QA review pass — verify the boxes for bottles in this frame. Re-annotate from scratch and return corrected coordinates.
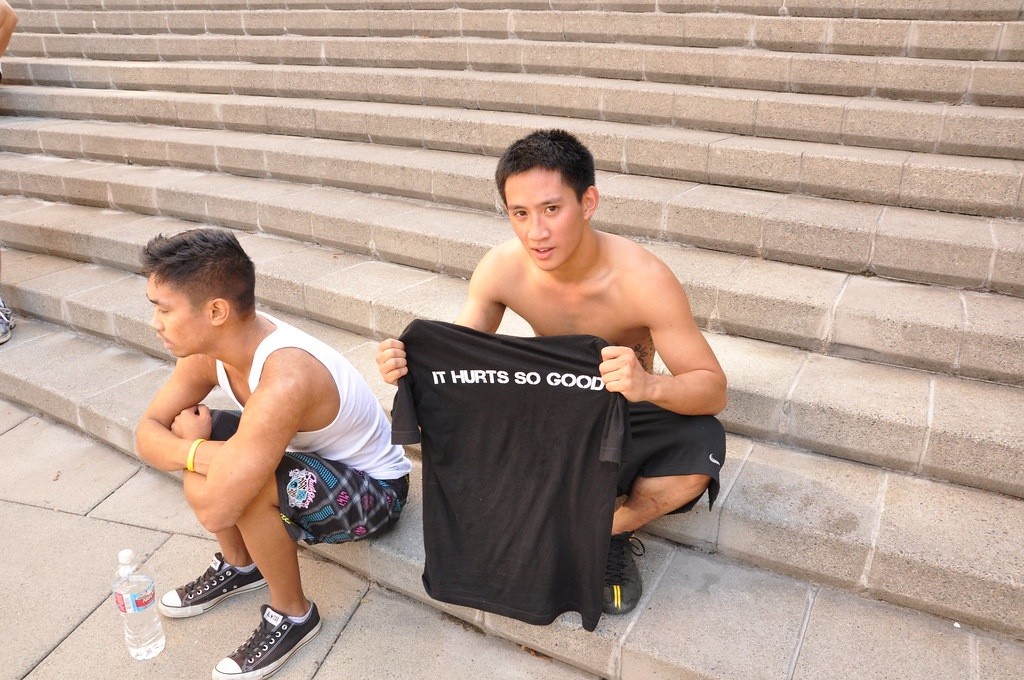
[115,548,164,660]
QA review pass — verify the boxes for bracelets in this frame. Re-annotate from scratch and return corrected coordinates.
[188,438,206,471]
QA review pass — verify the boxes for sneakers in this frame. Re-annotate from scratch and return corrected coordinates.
[212,601,321,680]
[159,552,268,618]
[601,530,645,615]
[0,297,15,344]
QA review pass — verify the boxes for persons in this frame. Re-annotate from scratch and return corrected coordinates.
[135,227,409,680]
[377,128,728,615]
[0,1,20,346]
